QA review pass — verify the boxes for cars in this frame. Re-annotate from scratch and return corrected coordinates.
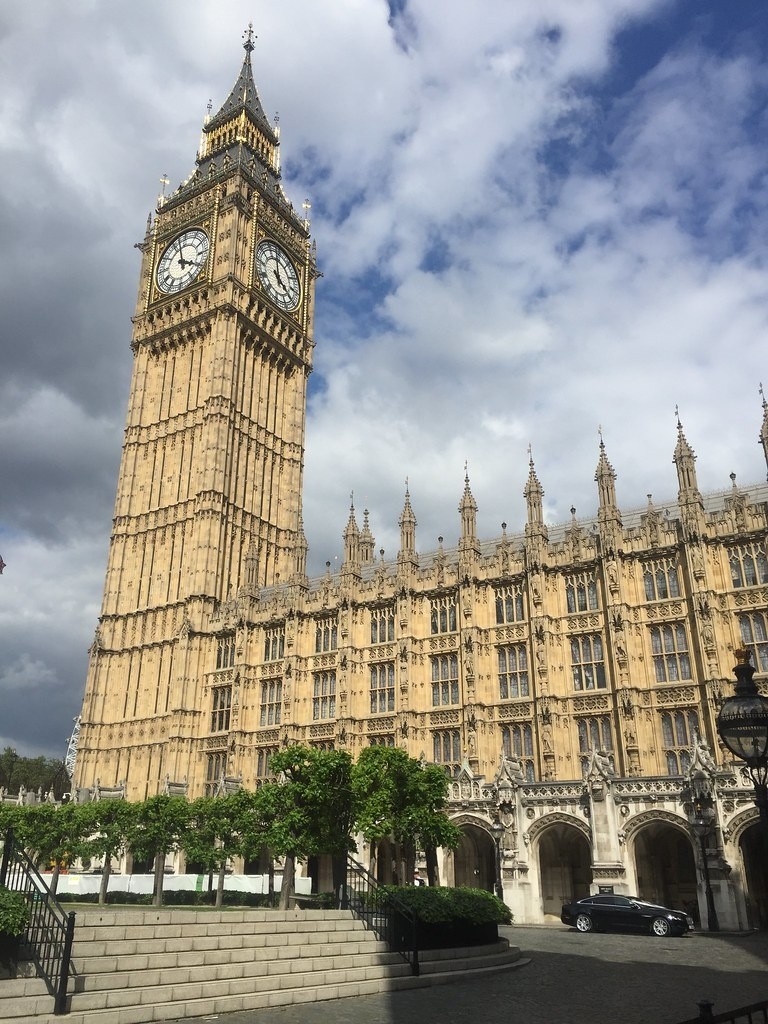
[559,893,695,939]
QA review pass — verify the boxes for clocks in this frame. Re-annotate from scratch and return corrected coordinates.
[153,226,210,294]
[254,236,302,313]
